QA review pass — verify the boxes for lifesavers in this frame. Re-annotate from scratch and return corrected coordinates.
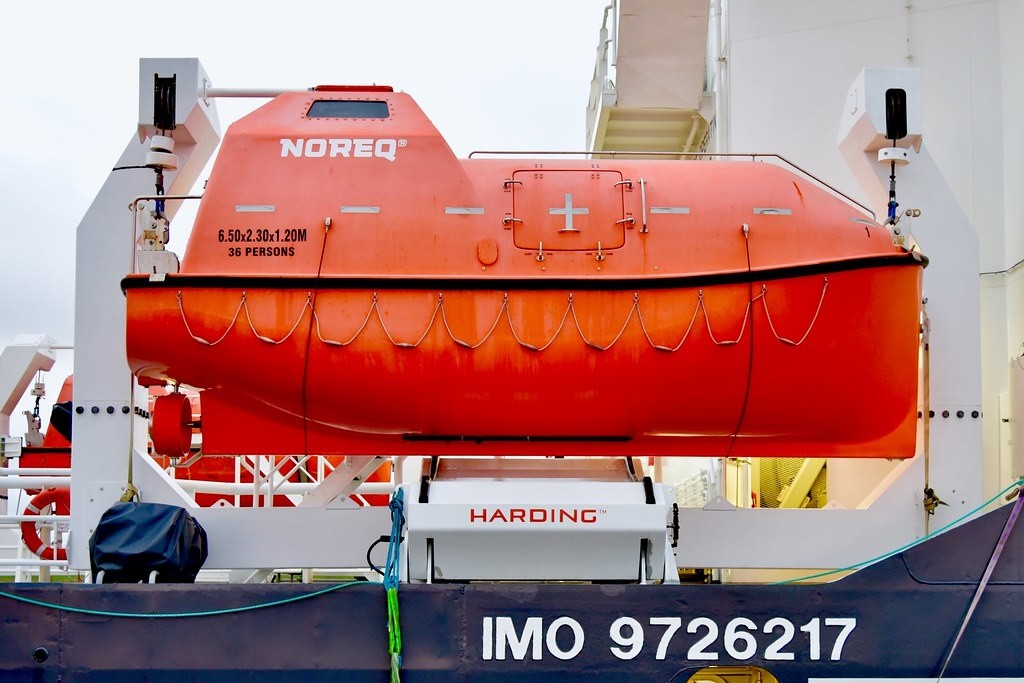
[21,489,70,560]
